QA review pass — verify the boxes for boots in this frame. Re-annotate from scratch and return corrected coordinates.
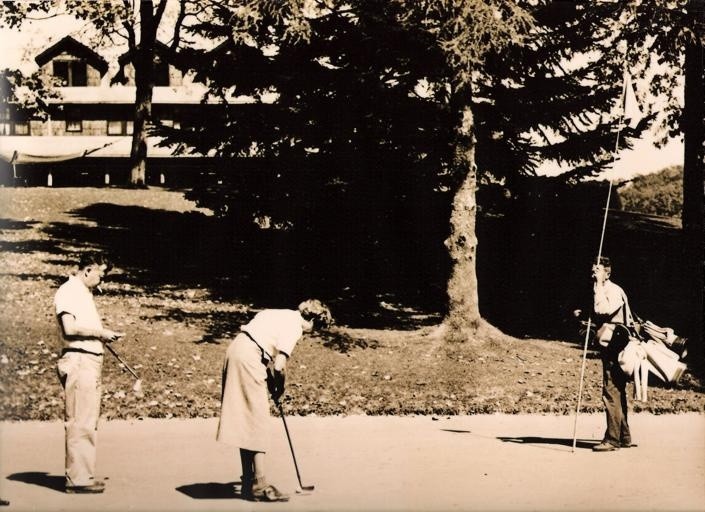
[240,475,291,502]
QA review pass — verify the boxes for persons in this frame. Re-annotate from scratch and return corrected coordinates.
[574,256,637,452]
[216,299,332,501]
[53,250,128,494]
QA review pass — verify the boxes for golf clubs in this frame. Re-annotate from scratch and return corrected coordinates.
[266,367,313,490]
[103,341,142,392]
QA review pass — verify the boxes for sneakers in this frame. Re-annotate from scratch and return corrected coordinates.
[66,480,105,493]
[592,442,631,451]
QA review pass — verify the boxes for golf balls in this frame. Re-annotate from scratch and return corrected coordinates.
[296,489,301,493]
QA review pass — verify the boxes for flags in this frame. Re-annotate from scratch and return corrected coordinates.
[622,76,643,121]
